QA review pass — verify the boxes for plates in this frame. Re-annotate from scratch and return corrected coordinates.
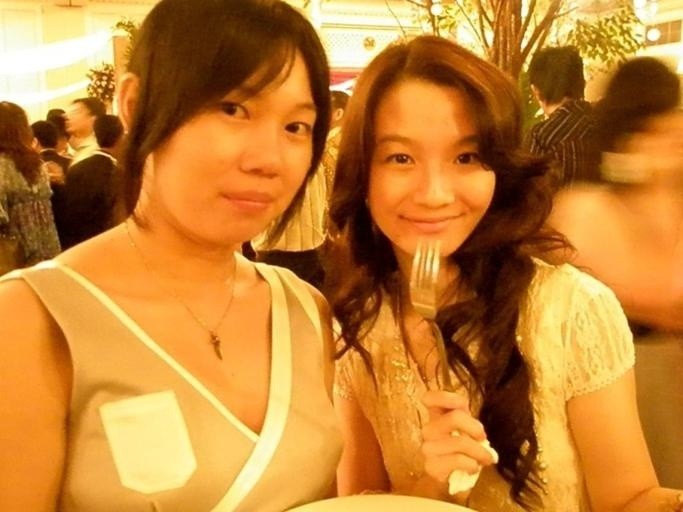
[288,495,476,512]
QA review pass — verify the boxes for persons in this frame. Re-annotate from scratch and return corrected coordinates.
[0,0,347,512]
[0,46,682,511]
[316,34,681,511]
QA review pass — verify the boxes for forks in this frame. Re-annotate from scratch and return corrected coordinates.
[407,237,455,396]
